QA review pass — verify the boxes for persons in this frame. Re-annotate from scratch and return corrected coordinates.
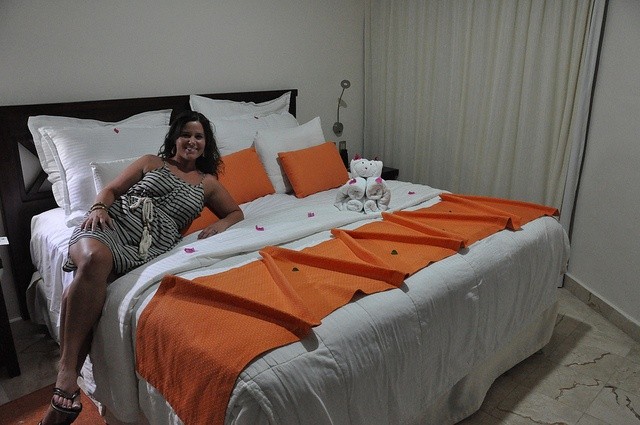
[38,111,244,425]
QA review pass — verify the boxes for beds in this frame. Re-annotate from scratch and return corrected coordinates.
[0,88,571,425]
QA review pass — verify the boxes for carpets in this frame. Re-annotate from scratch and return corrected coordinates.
[0,381,109,425]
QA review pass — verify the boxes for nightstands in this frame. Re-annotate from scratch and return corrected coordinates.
[1,251,25,383]
[343,160,402,182]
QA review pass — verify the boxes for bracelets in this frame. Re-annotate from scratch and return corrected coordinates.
[90,202,110,211]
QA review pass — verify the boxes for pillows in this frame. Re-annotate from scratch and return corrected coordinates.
[212,147,276,205]
[255,116,326,194]
[37,125,171,229]
[90,157,142,194]
[181,206,220,238]
[278,142,349,198]
[27,109,172,209]
[189,92,300,154]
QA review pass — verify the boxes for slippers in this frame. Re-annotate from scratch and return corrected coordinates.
[51,387,83,413]
